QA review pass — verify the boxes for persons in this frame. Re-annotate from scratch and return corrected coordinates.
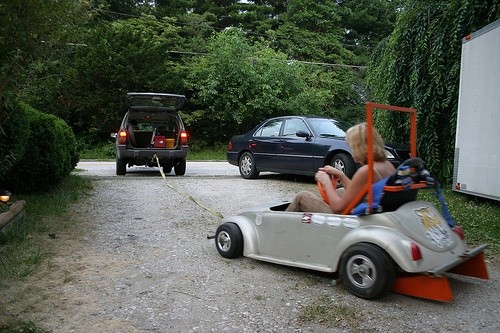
[285,121,399,217]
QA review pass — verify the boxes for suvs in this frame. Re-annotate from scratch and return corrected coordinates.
[111,92,188,176]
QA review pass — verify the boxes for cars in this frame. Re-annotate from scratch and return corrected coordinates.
[206,189,488,302]
[226,116,402,189]
